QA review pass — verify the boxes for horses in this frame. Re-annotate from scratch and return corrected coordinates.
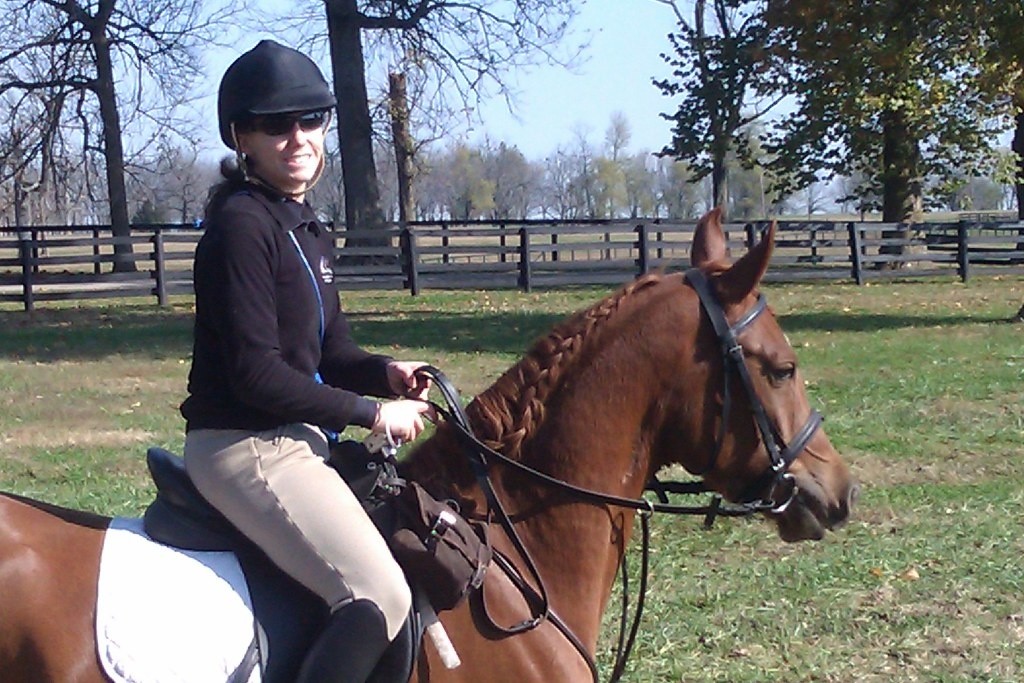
[0,207,857,683]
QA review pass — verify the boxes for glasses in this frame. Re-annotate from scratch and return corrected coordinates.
[234,106,331,137]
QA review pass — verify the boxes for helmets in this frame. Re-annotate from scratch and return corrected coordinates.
[216,39,338,152]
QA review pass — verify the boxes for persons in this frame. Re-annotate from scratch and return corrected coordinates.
[182,40,433,683]
